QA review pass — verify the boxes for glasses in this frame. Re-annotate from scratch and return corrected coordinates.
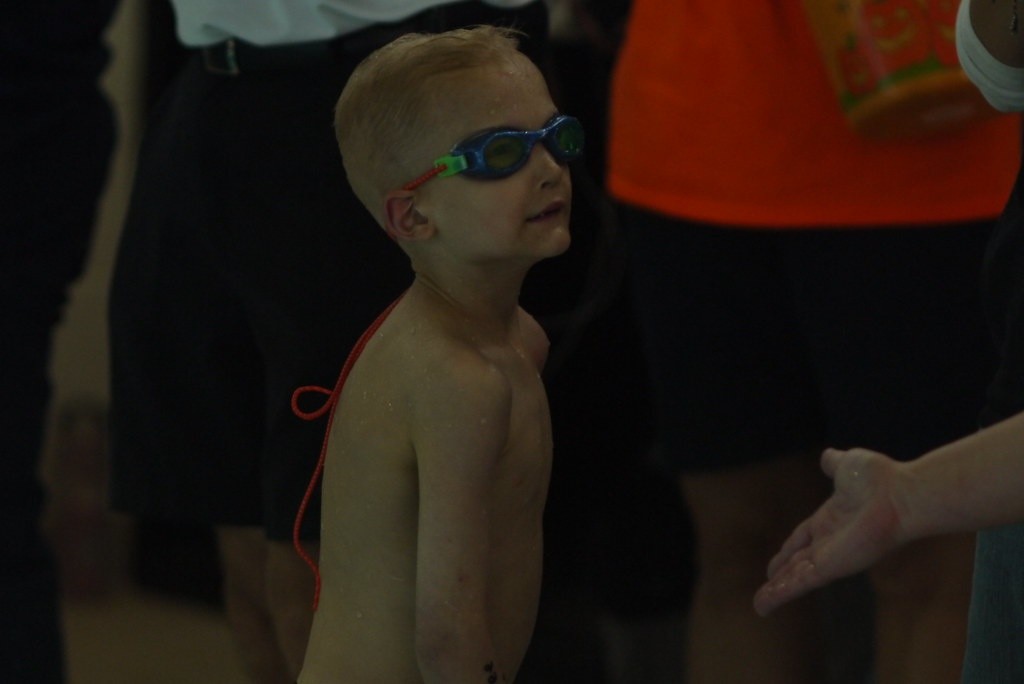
[400,112,588,192]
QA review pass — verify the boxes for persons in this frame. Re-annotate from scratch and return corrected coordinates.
[285,23,583,684]
[598,3,1023,684]
[1,1,551,684]
[751,0,1023,684]
